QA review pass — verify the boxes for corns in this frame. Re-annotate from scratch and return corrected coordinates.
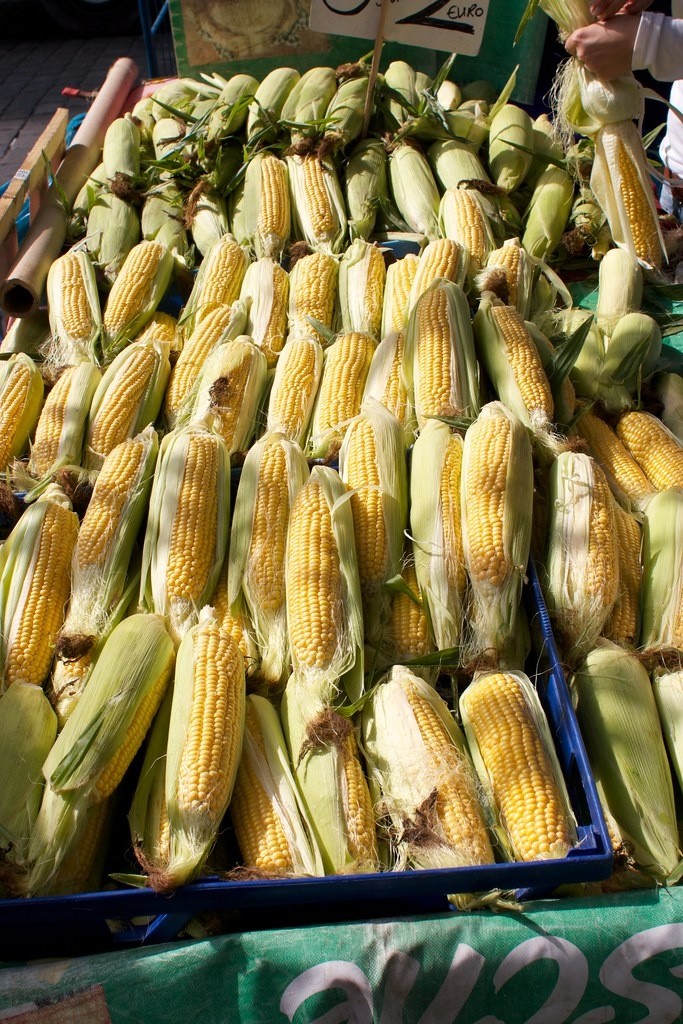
[0,64,683,894]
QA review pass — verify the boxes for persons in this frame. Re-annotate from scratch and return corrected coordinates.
[564,1,682,224]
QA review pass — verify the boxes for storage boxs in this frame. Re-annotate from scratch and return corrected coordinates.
[0,107,421,509]
[0,554,614,966]
